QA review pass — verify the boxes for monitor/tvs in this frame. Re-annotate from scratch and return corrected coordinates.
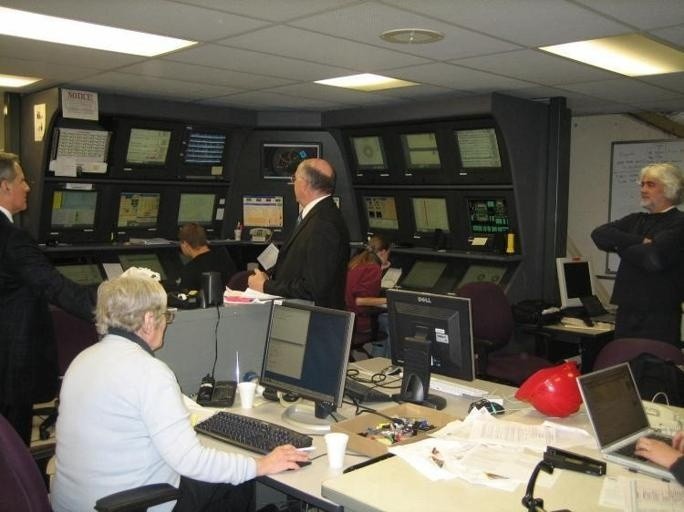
[241,193,285,229]
[556,255,597,309]
[258,298,356,431]
[387,289,475,410]
[50,124,228,288]
[349,128,519,294]
[260,142,323,182]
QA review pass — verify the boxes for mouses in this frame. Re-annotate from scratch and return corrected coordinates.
[382,365,402,375]
[287,461,312,471]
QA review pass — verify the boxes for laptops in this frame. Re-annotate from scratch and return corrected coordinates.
[575,361,676,479]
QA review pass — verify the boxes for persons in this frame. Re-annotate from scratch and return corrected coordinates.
[248,158,350,314]
[592,163,684,350]
[179,221,237,291]
[48,266,311,512]
[635,428,683,486]
[345,235,393,357]
[0,151,96,450]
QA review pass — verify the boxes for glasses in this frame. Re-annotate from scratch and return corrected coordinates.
[156,310,176,324]
[290,175,314,184]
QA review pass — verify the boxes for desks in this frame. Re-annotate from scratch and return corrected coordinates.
[179,354,539,512]
[321,393,684,512]
[516,316,615,374]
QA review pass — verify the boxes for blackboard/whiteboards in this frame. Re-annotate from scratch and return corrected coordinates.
[605,139,684,274]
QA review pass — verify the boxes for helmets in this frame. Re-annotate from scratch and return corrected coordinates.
[513,360,583,417]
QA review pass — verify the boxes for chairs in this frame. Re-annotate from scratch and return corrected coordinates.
[590,337,684,374]
[0,412,183,512]
[227,270,257,292]
[351,308,387,363]
[24,307,99,439]
[453,281,557,386]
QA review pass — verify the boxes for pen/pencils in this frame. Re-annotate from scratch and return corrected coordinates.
[627,468,670,482]
[236,221,243,229]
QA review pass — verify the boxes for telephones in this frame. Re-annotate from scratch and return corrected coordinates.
[250,227,272,243]
[197,373,238,407]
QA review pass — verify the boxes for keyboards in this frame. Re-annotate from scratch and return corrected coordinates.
[428,374,488,400]
[194,411,313,455]
[344,378,391,402]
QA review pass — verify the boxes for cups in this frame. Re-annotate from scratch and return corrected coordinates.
[237,382,258,413]
[234,230,243,240]
[325,432,350,472]
